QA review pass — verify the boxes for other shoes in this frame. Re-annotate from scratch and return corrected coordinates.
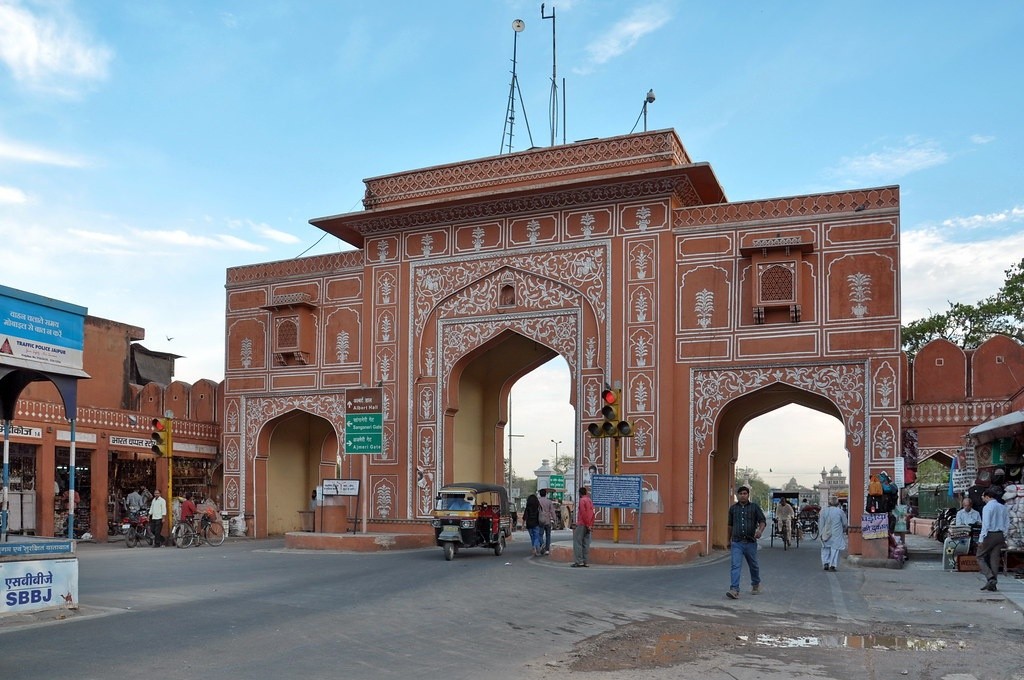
[536,553,541,556]
[545,551,549,555]
[751,585,758,595]
[829,566,836,571]
[532,547,536,553]
[570,563,589,567]
[980,580,997,592]
[726,591,739,599]
[824,564,829,570]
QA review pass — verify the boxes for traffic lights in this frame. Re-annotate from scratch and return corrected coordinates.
[587,388,635,439]
[151,418,168,458]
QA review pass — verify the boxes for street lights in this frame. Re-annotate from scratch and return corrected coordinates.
[551,439,562,466]
[508,18,526,153]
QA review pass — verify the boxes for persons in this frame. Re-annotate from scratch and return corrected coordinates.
[140,484,153,507]
[956,498,982,542]
[147,489,167,548]
[776,497,794,546]
[819,497,847,571]
[126,486,144,515]
[62,490,80,510]
[799,499,810,512]
[841,505,848,519]
[311,490,317,508]
[570,487,595,567]
[976,490,1010,591]
[176,492,206,548]
[449,494,472,510]
[538,490,557,554]
[726,487,766,599]
[521,494,542,556]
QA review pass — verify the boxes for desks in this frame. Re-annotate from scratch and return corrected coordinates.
[1000,550,1024,576]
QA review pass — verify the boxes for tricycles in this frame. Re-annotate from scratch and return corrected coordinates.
[770,491,800,550]
[799,504,821,540]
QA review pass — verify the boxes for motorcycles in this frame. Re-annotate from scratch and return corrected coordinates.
[123,508,154,549]
[431,482,511,561]
[936,507,956,543]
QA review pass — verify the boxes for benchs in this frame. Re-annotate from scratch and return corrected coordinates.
[472,505,501,534]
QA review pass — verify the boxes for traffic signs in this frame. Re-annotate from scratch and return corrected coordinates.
[345,414,383,433]
[346,389,383,414]
[345,434,383,455]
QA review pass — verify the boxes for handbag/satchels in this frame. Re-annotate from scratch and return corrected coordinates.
[538,505,546,526]
[821,518,832,542]
[866,473,898,513]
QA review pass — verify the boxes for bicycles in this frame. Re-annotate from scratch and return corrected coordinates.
[172,509,225,549]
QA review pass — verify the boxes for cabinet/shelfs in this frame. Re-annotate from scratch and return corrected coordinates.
[77,475,206,492]
